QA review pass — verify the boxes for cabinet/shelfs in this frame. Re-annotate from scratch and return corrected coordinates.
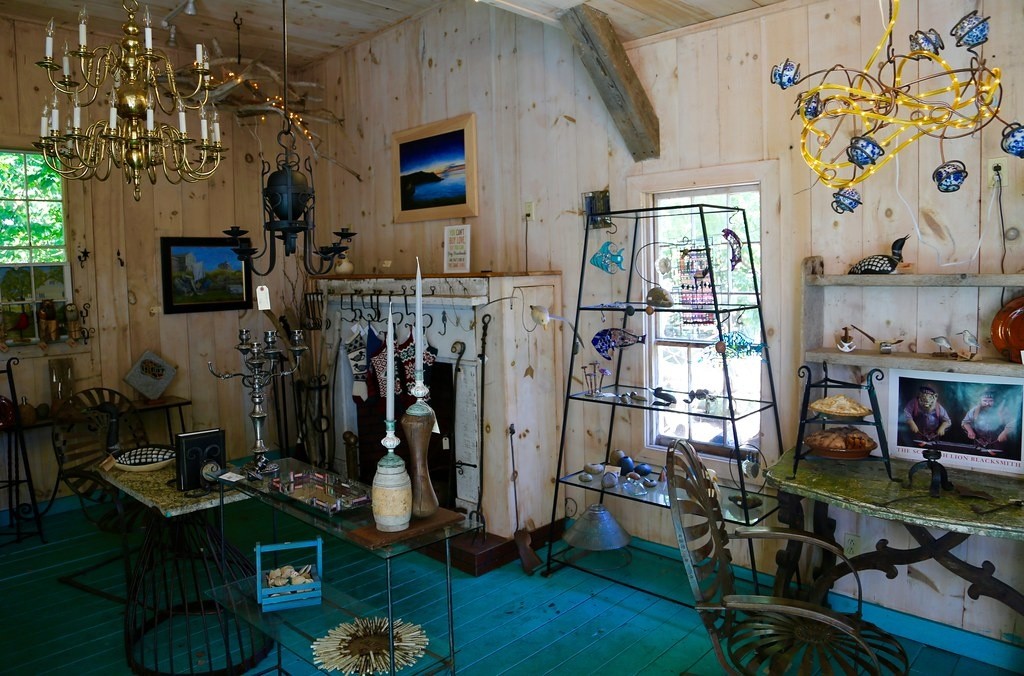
[204,457,484,676]
[798,252,1024,455]
[540,203,804,625]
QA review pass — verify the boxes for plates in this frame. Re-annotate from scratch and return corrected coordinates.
[110,444,176,471]
[5,338,41,346]
[802,440,878,460]
[808,405,874,421]
[990,296,1024,364]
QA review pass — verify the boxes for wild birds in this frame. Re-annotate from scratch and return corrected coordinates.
[849,233,911,275]
[955,329,982,353]
[930,335,954,353]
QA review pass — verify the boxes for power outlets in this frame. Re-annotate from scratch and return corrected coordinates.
[844,532,862,559]
[986,156,1009,188]
[525,202,535,222]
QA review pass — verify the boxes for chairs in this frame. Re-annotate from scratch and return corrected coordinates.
[49,387,261,615]
[666,438,910,676]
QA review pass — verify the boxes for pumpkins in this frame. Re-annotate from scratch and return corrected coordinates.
[19,396,36,426]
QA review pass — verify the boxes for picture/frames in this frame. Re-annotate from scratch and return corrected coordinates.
[159,235,253,315]
[390,112,479,225]
[890,367,1024,474]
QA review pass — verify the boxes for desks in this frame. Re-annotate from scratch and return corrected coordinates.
[762,443,1024,655]
[85,445,280,676]
[0,392,194,545]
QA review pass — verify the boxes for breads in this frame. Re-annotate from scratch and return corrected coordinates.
[805,425,876,451]
[809,395,873,416]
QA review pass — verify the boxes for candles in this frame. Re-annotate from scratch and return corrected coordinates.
[386,303,395,422]
[415,256,423,371]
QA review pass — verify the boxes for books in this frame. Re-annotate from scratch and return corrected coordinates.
[176,428,226,489]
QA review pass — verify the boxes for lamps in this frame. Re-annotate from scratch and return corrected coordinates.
[27,0,230,202]
[221,0,357,276]
